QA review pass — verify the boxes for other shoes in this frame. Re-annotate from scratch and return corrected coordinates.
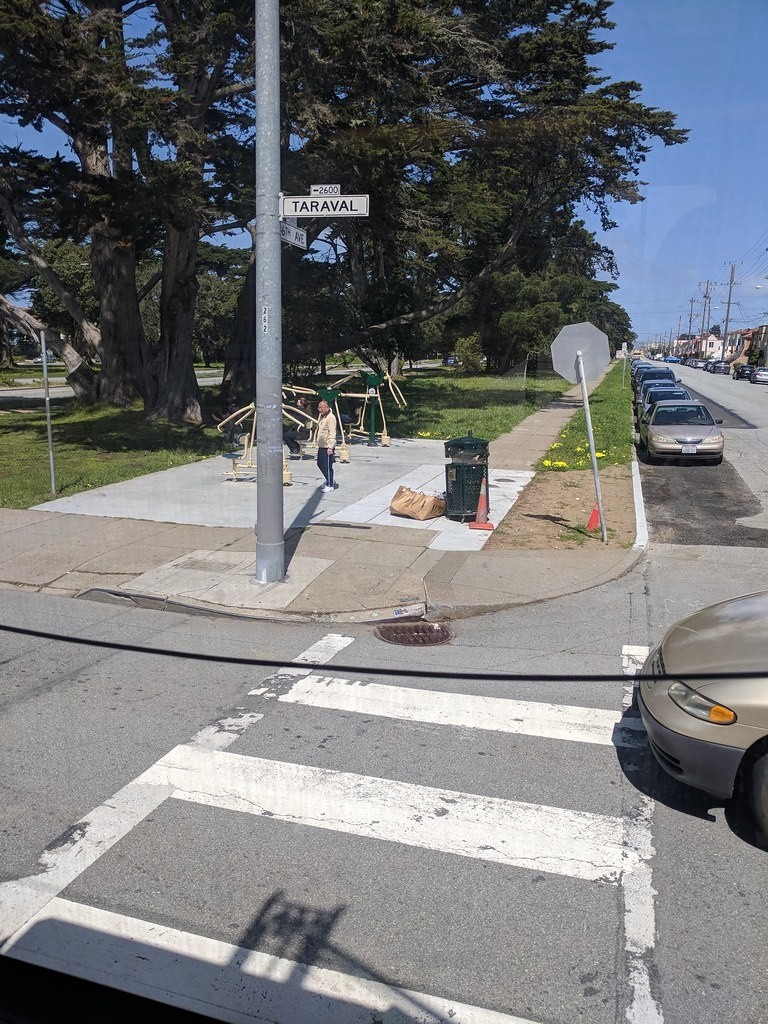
[288,447,301,454]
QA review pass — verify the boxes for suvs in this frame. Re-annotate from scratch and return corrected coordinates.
[665,357,681,364]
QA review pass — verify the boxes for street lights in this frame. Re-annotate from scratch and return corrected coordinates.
[696,321,709,359]
[715,307,730,360]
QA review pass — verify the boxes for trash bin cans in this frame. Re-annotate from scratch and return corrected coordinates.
[443,437,490,524]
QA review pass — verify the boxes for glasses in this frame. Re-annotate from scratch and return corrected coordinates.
[317,406,328,411]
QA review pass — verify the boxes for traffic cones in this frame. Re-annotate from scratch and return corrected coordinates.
[469,478,494,530]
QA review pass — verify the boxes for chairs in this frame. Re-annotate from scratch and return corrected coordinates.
[342,406,362,439]
[294,420,314,460]
[223,433,250,482]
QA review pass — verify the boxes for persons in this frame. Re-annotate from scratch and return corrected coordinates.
[284,398,311,454]
[224,401,246,446]
[317,402,337,492]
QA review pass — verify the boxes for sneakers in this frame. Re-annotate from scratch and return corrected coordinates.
[320,486,335,493]
[319,481,327,489]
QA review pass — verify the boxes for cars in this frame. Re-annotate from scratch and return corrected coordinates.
[751,367,768,384]
[630,353,700,433]
[710,360,731,375]
[733,365,756,380]
[442,357,458,367]
[640,400,724,464]
[638,590,768,852]
[685,359,715,372]
[33,354,56,364]
